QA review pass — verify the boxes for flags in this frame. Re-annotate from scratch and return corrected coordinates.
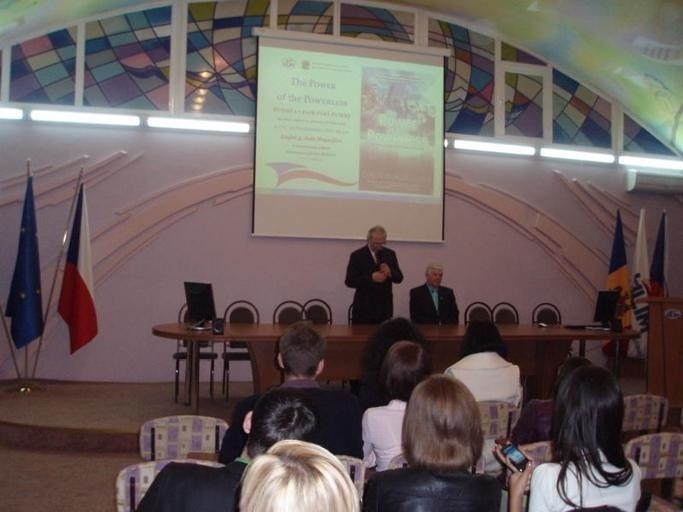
[649,211,665,295]
[627,209,648,358]
[607,209,632,332]
[56,182,98,356]
[3,175,42,350]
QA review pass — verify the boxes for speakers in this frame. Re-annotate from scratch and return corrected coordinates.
[212,317,225,336]
[611,319,623,333]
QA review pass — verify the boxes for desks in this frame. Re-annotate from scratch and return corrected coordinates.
[152,324,641,400]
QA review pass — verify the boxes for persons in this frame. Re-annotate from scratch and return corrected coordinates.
[133,386,320,512]
[495,364,642,512]
[443,315,523,411]
[219,320,363,465]
[345,224,403,323]
[236,440,361,512]
[363,374,502,512]
[360,340,434,471]
[510,357,592,443]
[408,262,460,325]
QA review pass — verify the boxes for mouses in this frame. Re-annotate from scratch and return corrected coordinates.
[539,322,548,328]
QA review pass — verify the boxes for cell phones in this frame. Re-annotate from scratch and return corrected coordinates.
[500,444,529,471]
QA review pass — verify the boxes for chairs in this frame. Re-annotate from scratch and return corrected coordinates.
[464,302,493,324]
[342,303,353,388]
[173,302,217,405]
[300,298,332,384]
[532,302,561,324]
[222,300,259,401]
[491,302,520,324]
[273,300,308,324]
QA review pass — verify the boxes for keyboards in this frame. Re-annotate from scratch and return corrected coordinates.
[564,324,602,329]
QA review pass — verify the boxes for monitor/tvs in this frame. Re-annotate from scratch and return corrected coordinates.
[592,290,620,328]
[183,281,216,330]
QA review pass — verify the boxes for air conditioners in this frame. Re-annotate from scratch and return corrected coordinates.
[625,169,683,194]
